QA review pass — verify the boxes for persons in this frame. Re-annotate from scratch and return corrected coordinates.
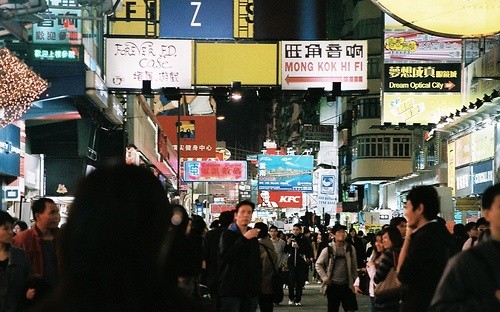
[21,164,210,312]
[314,224,363,312]
[301,226,313,285]
[267,225,286,307]
[315,233,330,284]
[372,225,407,312]
[460,216,490,252]
[254,221,279,312]
[165,203,190,292]
[185,91,218,116]
[396,183,458,311]
[283,232,290,290]
[389,216,408,241]
[13,220,29,236]
[278,229,285,239]
[283,223,314,306]
[200,210,234,311]
[182,213,206,301]
[430,183,500,311]
[208,219,220,231]
[348,227,362,291]
[215,199,263,311]
[352,231,386,311]
[466,220,477,236]
[258,191,278,208]
[381,222,390,228]
[310,231,323,284]
[356,229,368,254]
[1,210,34,312]
[12,196,65,281]
[451,223,468,243]
[446,219,456,235]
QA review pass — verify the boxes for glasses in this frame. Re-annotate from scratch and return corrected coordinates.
[478,228,487,232]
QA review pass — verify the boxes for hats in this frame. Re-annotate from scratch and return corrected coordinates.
[254,222,268,240]
[302,227,311,234]
[332,223,347,234]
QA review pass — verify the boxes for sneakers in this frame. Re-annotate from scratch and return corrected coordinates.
[296,302,301,306]
[288,299,294,306]
[305,281,309,285]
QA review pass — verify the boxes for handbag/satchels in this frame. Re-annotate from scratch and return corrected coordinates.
[373,250,402,295]
[357,268,370,296]
[272,272,284,304]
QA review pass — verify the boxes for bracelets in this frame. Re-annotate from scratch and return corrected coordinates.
[403,236,410,244]
